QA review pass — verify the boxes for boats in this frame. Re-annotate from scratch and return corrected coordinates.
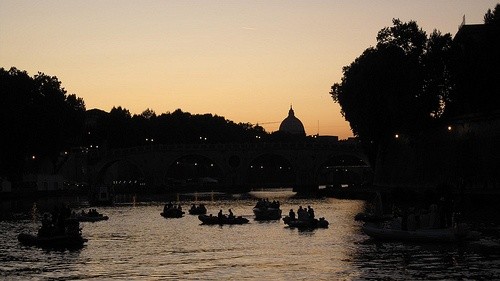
[363,222,462,241]
[188,208,207,215]
[198,214,249,224]
[78,213,103,221]
[160,210,183,218]
[252,207,281,219]
[283,217,329,229]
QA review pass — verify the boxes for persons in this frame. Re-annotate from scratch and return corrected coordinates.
[227,208,233,219]
[191,204,205,211]
[297,205,315,221]
[254,198,280,209]
[81,208,99,217]
[164,200,182,212]
[37,215,80,238]
[218,209,222,217]
[451,212,470,232]
[391,208,420,232]
[289,209,295,219]
